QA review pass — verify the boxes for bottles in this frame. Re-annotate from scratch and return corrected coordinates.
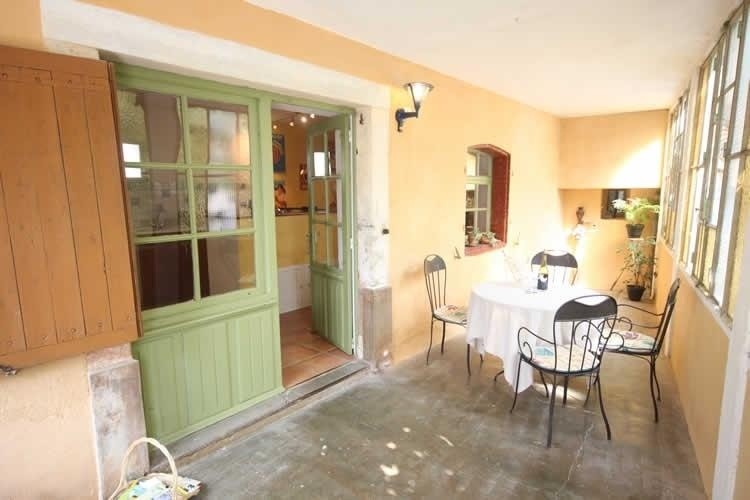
[537,253,548,290]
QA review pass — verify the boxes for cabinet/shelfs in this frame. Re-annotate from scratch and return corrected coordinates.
[277,264,312,314]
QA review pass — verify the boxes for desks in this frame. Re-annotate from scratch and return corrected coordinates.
[467,278,605,393]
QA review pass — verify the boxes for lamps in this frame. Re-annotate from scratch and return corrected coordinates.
[396,81,435,131]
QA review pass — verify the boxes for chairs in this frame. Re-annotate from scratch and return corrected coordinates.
[594,278,681,424]
[510,295,617,447]
[423,253,483,376]
[530,248,578,286]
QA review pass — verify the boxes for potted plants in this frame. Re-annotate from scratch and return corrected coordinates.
[480,232,496,243]
[616,238,658,301]
[612,198,661,238]
